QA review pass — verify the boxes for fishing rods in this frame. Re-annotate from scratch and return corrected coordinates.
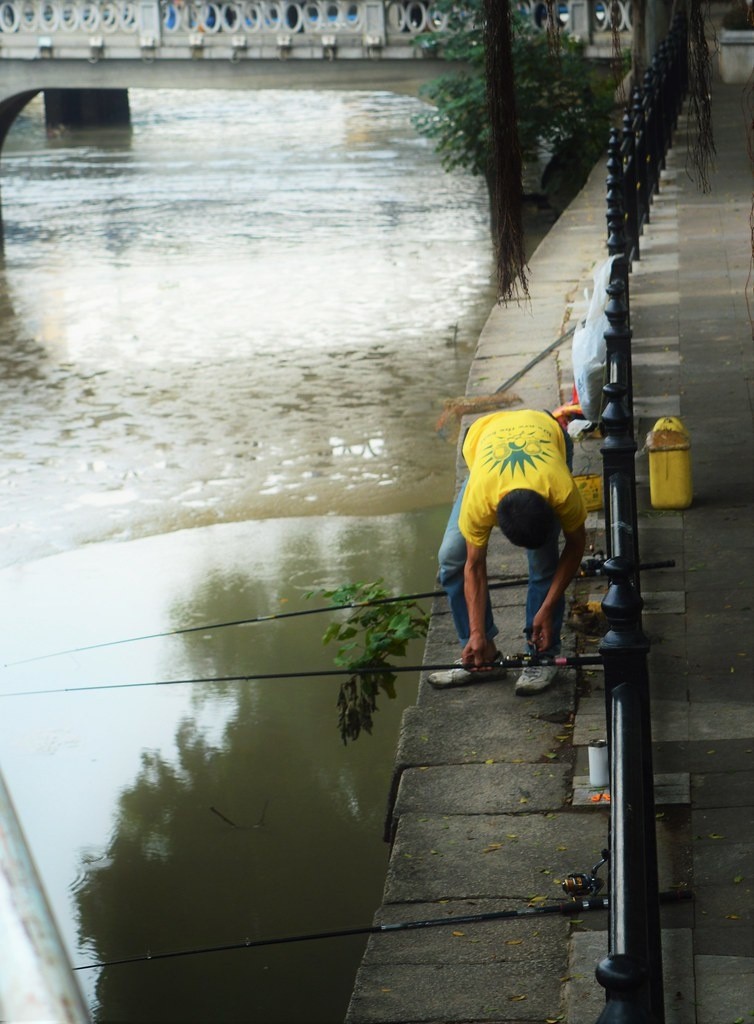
[70,850,696,973]
[0,560,678,669]
[0,651,603,698]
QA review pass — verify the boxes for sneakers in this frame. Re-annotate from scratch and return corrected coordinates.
[514,665,559,693]
[427,650,506,688]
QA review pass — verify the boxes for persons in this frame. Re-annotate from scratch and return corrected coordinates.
[421,409,589,697]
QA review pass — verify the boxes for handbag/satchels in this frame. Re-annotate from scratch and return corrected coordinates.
[571,253,625,423]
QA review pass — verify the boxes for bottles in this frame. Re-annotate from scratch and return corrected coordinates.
[649,416,693,510]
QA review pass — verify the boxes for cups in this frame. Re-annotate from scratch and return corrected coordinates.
[588,739,609,787]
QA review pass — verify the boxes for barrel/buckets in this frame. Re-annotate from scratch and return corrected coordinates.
[573,466,602,511]
